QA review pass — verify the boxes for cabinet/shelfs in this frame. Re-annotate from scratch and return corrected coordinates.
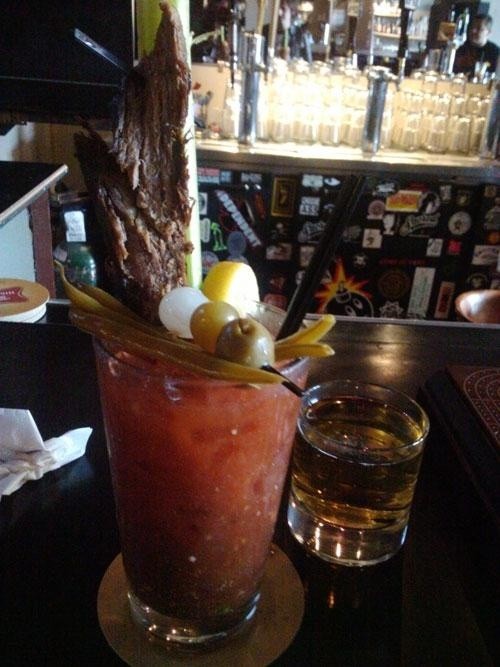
[356,0,429,53]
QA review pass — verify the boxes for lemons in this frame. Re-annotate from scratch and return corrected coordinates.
[203,260,260,302]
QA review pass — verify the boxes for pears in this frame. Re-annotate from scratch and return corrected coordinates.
[215,318,274,368]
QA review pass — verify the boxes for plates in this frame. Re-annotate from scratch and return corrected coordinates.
[97,544,309,667]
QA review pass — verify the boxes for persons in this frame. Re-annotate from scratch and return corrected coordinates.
[258,0,313,68]
[449,288,500,325]
[450,14,499,82]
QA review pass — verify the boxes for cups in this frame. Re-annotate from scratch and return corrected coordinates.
[288,380,430,571]
[88,332,313,652]
[221,54,493,155]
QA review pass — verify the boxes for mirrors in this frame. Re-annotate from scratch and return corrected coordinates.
[189,0,500,84]
